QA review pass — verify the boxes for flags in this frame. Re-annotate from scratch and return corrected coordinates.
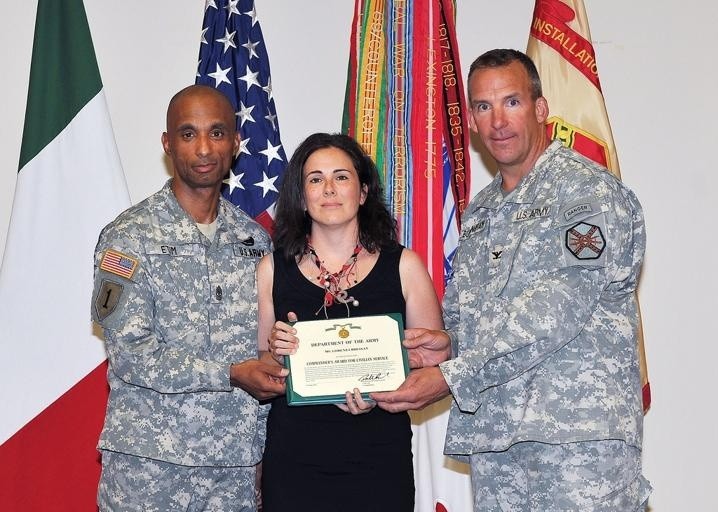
[442,0,650,475]
[340,0,472,427]
[195,1,289,237]
[1,1,135,511]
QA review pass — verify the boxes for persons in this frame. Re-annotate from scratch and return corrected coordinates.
[366,50,653,511]
[90,86,293,512]
[257,134,446,511]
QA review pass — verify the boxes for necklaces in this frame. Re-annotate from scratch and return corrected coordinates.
[305,238,365,319]
